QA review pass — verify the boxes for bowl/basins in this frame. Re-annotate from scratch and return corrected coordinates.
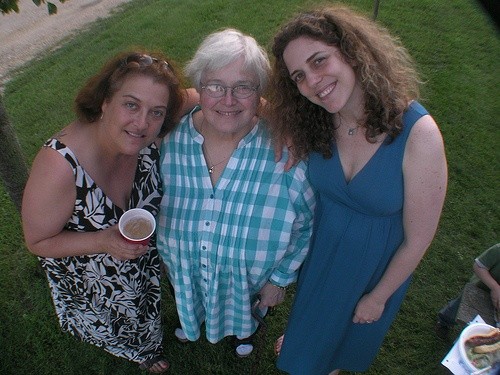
[459,323,500,375]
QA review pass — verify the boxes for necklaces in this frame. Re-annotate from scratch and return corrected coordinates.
[199,118,232,174]
[339,116,367,135]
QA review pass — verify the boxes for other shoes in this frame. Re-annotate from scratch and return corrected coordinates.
[436,318,451,340]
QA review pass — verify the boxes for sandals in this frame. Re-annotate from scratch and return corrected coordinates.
[175,324,187,339]
[234,337,254,359]
[143,357,170,374]
[274,334,285,357]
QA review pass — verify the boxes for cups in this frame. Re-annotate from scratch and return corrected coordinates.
[118,208,156,246]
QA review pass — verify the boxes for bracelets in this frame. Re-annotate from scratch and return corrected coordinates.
[272,284,290,291]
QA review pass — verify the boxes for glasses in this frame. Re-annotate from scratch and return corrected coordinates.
[199,79,261,100]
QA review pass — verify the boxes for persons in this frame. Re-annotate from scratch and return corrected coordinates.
[19,49,311,375]
[155,27,318,360]
[434,243,500,342]
[262,5,449,375]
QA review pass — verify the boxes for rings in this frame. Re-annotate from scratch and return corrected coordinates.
[366,321,370,324]
